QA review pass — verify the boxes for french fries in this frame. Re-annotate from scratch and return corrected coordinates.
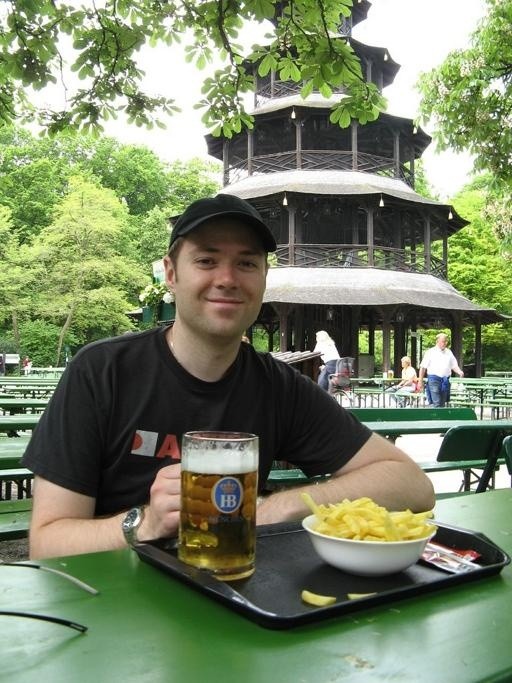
[347,592,377,600]
[302,493,438,543]
[301,590,336,607]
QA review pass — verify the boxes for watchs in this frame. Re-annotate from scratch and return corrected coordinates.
[121,503,151,546]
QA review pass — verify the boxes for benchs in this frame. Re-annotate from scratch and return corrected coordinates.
[3,497,32,544]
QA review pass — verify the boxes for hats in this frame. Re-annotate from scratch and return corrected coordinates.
[169,195,277,251]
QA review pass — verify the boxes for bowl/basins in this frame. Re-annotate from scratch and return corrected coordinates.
[302,514,438,578]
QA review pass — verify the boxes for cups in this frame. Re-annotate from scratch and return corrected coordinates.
[177,431,260,583]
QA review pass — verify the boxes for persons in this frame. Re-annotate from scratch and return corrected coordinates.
[415,331,465,438]
[385,356,418,407]
[18,193,438,561]
[24,355,30,376]
[310,329,341,392]
